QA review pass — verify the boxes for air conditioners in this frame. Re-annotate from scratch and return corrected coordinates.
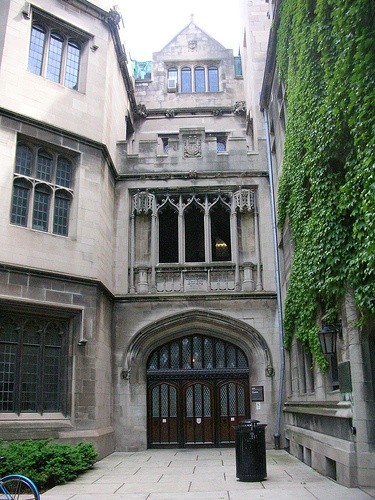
[168,77,177,93]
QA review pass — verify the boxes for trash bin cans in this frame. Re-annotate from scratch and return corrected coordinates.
[233,420,267,482]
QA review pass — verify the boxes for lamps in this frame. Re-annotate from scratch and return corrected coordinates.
[318,319,343,355]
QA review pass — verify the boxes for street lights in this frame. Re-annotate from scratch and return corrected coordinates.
[318,327,340,391]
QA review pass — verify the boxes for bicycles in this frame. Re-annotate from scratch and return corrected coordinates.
[0,456,40,500]
